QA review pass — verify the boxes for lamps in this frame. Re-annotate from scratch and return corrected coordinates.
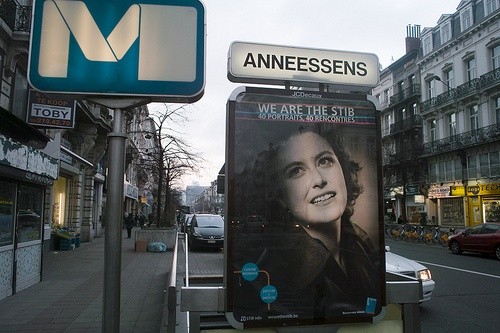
[138,151,152,156]
[130,130,152,139]
[132,147,148,152]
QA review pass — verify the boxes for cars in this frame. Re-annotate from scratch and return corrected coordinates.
[446,222,500,259]
[189,214,225,251]
[385,245,436,304]
[181,214,195,234]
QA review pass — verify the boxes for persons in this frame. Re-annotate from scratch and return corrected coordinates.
[124,209,154,238]
[177,212,180,226]
[226,122,378,323]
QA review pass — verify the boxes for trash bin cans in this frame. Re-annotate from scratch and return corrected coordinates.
[57,229,79,251]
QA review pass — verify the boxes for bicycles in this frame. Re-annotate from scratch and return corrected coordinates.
[386,222,461,247]
